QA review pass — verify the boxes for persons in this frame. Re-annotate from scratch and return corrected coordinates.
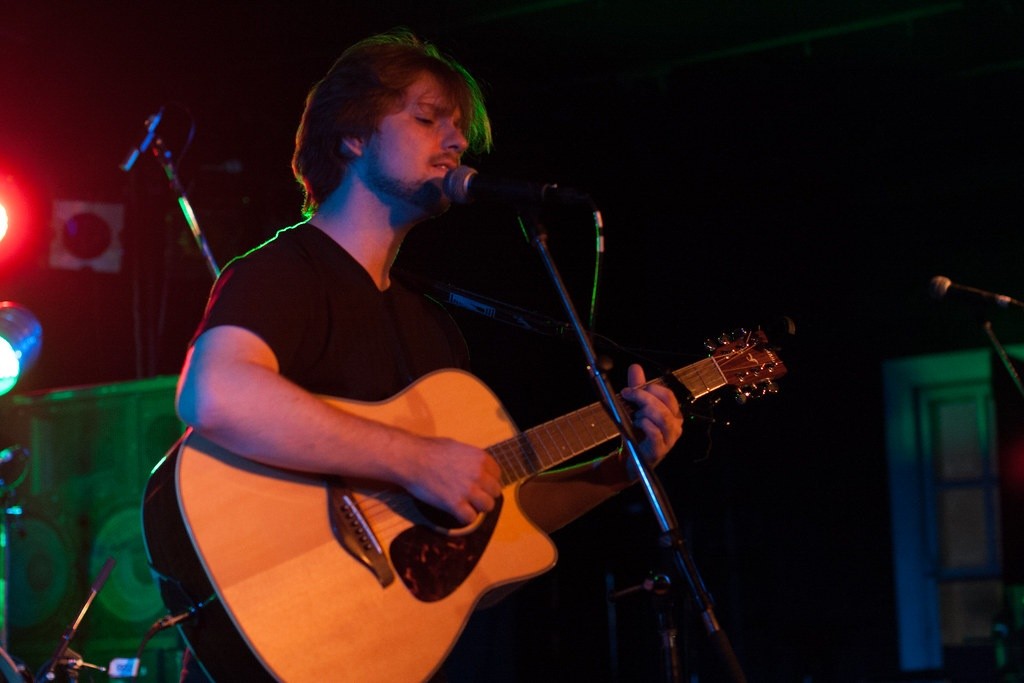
[174,32,688,683]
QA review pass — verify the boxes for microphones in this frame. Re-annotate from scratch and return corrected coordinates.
[444,168,592,207]
[119,106,166,171]
[929,275,1024,309]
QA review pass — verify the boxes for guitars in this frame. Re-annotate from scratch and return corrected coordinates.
[139,323,791,683]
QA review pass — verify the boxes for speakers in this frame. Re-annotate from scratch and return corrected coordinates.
[4,376,189,683]
